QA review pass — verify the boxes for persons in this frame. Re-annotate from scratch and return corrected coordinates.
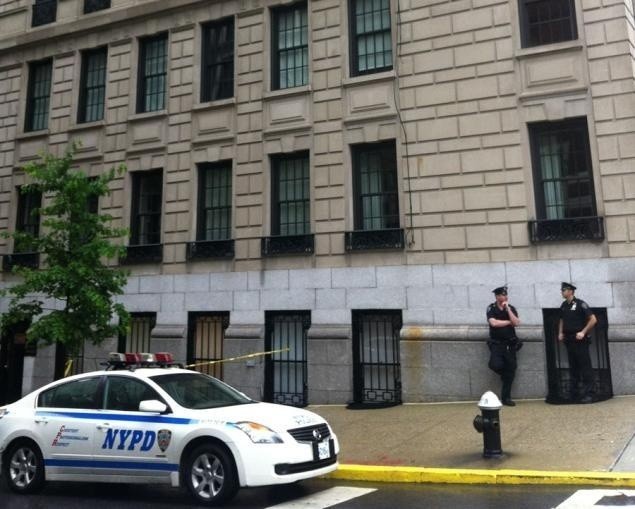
[485,286,521,406]
[558,281,597,403]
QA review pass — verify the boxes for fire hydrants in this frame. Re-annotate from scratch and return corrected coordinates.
[473,390,502,458]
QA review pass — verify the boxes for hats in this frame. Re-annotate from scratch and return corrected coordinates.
[493,287,508,295]
[561,282,576,291]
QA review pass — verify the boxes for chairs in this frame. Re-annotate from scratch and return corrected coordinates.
[55,393,99,408]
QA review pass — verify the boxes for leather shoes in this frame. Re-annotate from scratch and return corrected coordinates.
[581,395,592,402]
[502,400,515,406]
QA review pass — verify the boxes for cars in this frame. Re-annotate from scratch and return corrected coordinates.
[1,351,340,505]
